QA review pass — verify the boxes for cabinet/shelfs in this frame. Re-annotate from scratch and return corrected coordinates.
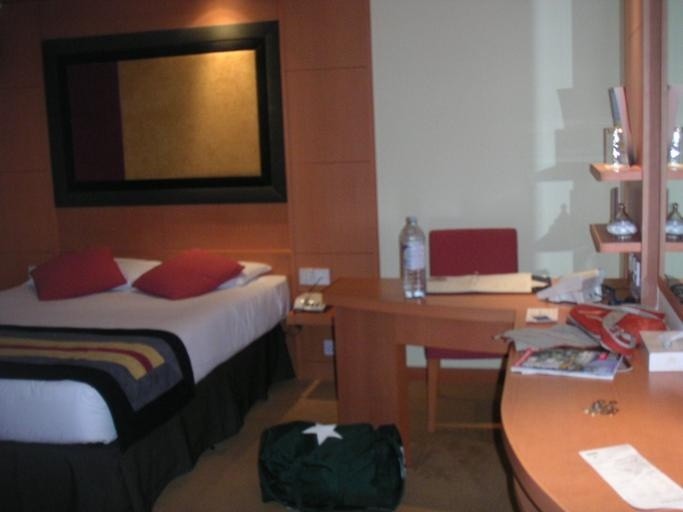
[665,165,682,251]
[589,163,642,255]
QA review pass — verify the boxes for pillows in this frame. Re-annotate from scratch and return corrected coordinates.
[127,258,272,290]
[130,250,244,300]
[25,240,126,300]
[28,257,148,292]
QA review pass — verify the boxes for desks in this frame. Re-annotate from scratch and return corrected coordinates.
[325,272,683,511]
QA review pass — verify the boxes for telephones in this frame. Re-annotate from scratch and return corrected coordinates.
[293,292,327,312]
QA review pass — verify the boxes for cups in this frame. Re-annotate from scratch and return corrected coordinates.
[603,129,623,170]
[667,128,682,166]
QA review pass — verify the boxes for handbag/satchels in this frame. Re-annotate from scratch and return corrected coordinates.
[492,301,668,353]
[259,421,405,512]
[427,273,551,294]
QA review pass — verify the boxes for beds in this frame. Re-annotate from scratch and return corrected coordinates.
[0,241,293,511]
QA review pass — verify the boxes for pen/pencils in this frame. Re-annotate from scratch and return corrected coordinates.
[514,347,534,367]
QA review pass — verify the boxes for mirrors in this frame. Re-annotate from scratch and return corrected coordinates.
[640,1,683,330]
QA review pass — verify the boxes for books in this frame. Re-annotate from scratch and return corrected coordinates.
[510,347,633,381]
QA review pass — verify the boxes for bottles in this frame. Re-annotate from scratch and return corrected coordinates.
[606,202,637,236]
[664,203,683,239]
[400,215,427,299]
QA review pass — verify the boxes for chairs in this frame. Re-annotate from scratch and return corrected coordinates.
[426,226,522,437]
